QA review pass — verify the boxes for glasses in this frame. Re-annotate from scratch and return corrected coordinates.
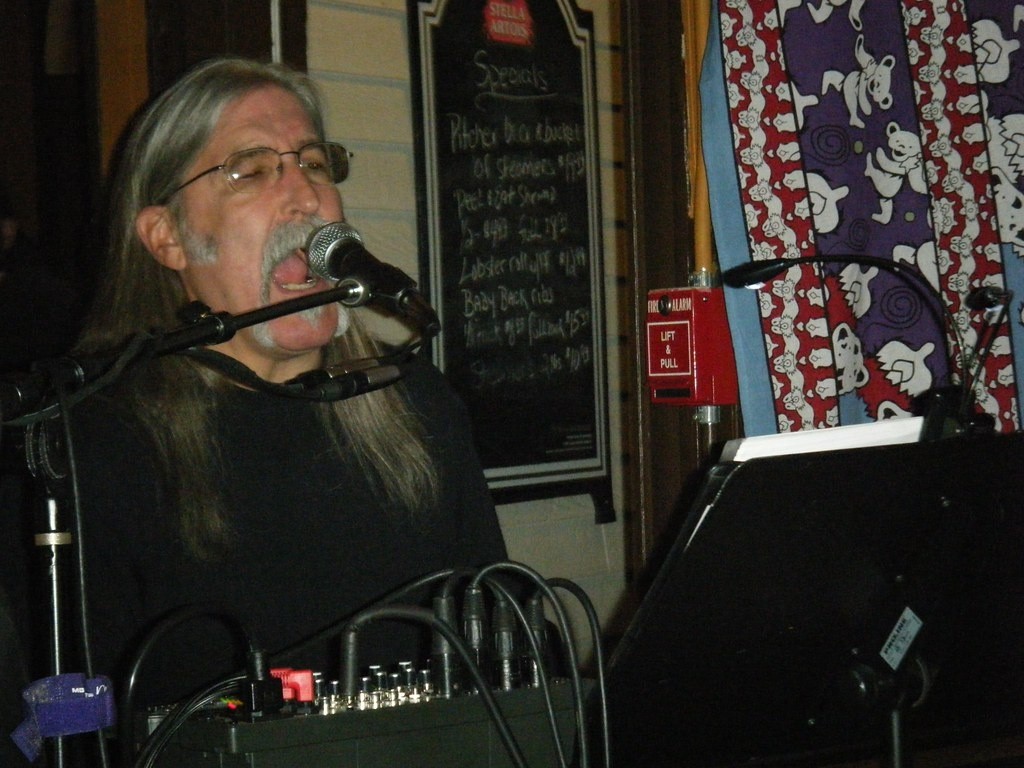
[156,141,350,192]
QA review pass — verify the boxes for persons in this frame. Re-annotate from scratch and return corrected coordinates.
[32,59,510,709]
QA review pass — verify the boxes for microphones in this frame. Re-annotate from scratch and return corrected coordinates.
[307,221,441,338]
[723,253,995,440]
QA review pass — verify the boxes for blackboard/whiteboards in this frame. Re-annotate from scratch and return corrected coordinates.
[408,0,614,490]
